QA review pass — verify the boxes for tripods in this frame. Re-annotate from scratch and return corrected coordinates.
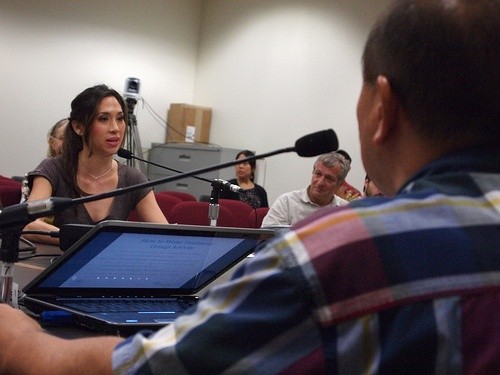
[121,115,148,172]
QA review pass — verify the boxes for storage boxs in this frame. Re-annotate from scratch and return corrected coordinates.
[165,102,212,144]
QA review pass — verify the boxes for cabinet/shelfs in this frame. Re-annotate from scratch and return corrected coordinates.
[148,142,255,201]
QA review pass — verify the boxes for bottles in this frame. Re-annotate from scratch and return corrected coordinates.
[19,173,31,205]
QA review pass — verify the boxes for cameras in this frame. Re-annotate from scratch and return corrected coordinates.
[125,78,140,93]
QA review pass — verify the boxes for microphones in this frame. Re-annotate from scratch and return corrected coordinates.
[0,129,339,226]
[117,147,243,193]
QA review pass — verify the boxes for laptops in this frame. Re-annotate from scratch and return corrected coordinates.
[23,218,274,331]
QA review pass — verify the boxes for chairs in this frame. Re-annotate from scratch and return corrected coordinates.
[0,175,269,228]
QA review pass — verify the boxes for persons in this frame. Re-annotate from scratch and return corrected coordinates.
[21,84,169,246]
[218,150,269,209]
[48,119,69,158]
[261,151,351,228]
[363,175,384,198]
[0,0,500,375]
[335,149,362,201]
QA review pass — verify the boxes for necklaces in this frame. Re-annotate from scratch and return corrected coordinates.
[78,158,112,179]
